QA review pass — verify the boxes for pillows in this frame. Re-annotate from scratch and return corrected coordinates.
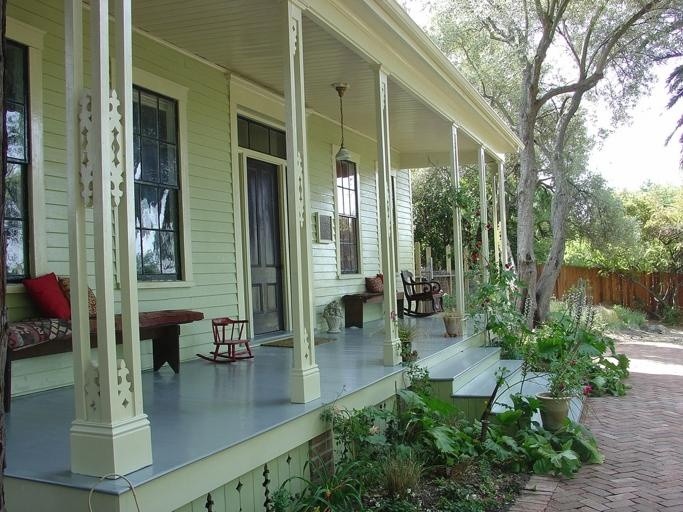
[5,272,99,352]
[365,274,384,293]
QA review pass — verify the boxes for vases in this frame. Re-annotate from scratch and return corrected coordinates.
[325,316,341,333]
[535,393,569,429]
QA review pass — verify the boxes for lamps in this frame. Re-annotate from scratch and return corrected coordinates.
[331,81,349,161]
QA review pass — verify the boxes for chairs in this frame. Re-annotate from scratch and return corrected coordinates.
[196,317,253,363]
[401,270,440,318]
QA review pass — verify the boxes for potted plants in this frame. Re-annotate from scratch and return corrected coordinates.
[397,320,416,362]
[442,294,461,335]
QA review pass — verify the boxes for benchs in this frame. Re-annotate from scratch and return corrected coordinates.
[340,291,403,327]
[5,310,204,409]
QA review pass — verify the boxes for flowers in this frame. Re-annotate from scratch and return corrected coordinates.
[546,351,596,398]
[322,299,344,317]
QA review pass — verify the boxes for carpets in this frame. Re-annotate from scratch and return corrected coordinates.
[260,335,337,349]
